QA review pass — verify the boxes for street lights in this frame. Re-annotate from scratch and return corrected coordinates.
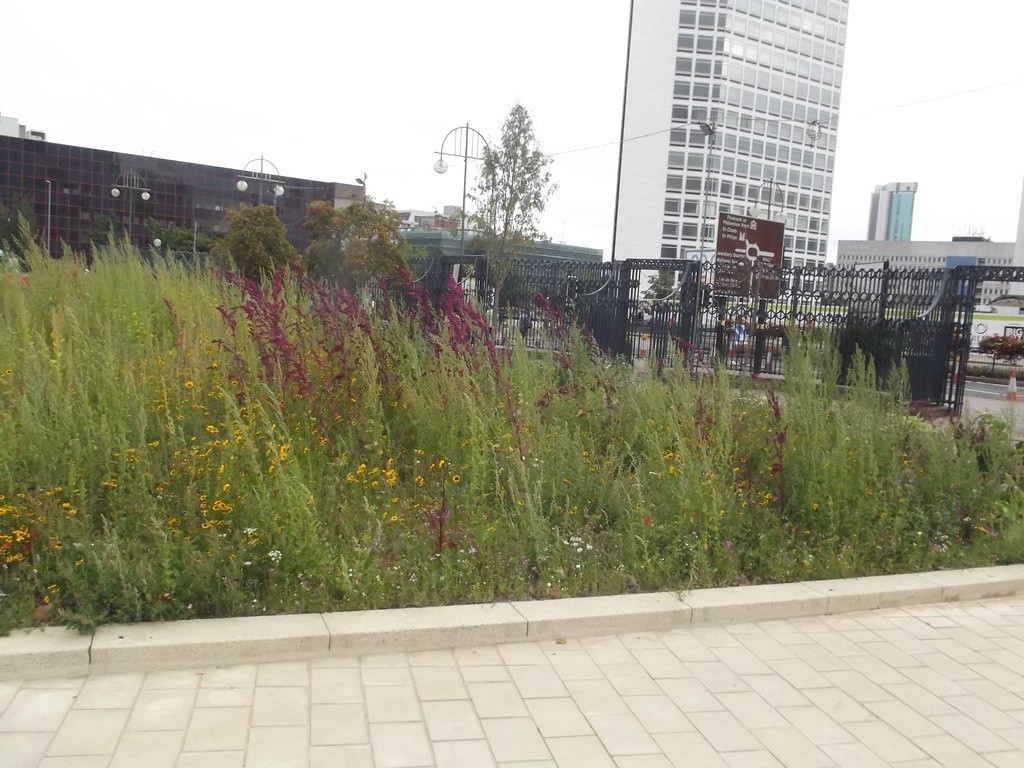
[432,122,495,256]
[236,154,286,205]
[112,168,151,243]
[42,179,51,255]
[750,178,787,222]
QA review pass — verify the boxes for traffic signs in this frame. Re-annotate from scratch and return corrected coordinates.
[713,212,785,299]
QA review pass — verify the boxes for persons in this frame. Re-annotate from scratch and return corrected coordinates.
[519,313,529,340]
[486,305,499,336]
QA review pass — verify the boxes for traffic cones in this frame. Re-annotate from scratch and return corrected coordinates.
[640,335,648,358]
[954,363,959,383]
[728,336,735,357]
[766,346,774,373]
[1006,369,1017,402]
